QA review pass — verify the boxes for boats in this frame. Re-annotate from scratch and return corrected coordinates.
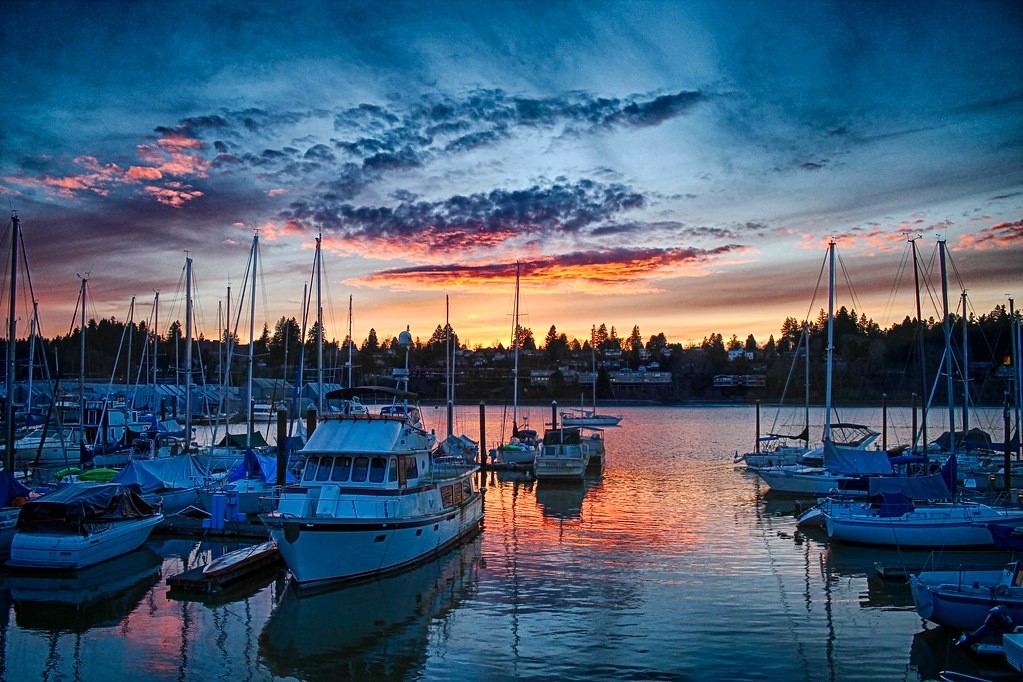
[202,542,278,575]
[253,537,486,682]
[532,400,590,479]
[934,605,1023,682]
[254,322,487,585]
[907,526,1023,635]
[509,415,542,447]
[578,426,606,468]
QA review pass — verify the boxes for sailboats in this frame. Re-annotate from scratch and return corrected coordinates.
[496,259,539,465]
[561,323,623,426]
[733,237,1023,547]
[0,545,166,682]
[0,214,479,570]
